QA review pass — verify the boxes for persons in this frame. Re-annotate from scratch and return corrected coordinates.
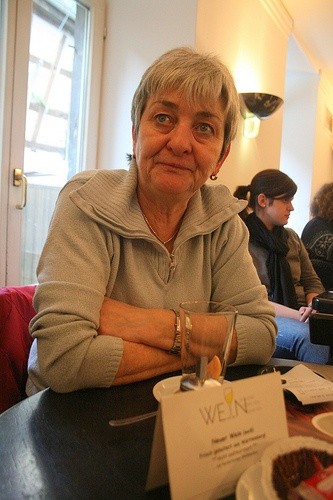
[233,169,333,365]
[25,47,278,398]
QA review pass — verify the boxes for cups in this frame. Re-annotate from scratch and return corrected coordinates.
[179,300,237,392]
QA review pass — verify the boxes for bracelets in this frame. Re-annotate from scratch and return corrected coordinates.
[307,303,312,307]
[167,308,192,355]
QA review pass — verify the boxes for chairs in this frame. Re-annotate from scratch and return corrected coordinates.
[0,285,37,414]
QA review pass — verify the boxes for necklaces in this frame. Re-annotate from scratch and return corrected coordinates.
[142,208,179,246]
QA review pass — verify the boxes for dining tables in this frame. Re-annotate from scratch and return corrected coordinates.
[0,359,333,500]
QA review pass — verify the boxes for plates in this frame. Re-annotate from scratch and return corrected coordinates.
[312,412,333,439]
[236,462,269,500]
[152,375,233,405]
